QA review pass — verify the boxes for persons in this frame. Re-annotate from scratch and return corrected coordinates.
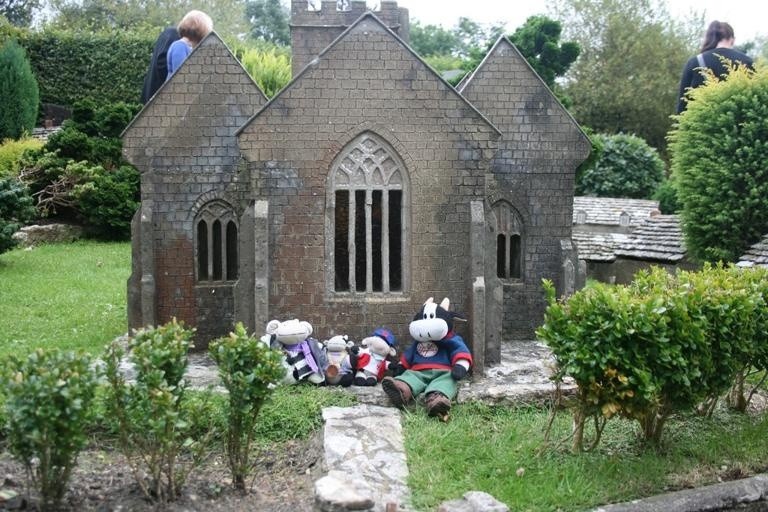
[139,28,181,105]
[166,10,213,77]
[676,20,754,114]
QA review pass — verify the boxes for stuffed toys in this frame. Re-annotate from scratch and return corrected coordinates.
[382,297,473,417]
[258,319,328,389]
[319,334,354,387]
[351,326,398,387]
